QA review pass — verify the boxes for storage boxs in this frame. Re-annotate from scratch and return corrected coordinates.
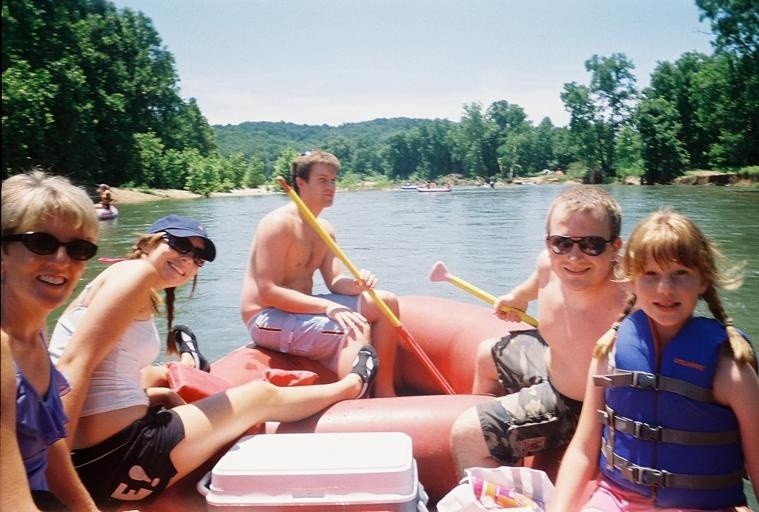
[197,432,431,512]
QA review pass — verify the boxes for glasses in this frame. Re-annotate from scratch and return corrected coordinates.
[162,234,205,266]
[2,231,97,260]
[547,235,611,256]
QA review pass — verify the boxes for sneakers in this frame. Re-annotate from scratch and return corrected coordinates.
[352,344,377,399]
[173,325,210,372]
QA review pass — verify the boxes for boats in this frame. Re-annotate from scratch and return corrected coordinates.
[89,294,756,512]
[89,199,121,224]
[400,184,451,193]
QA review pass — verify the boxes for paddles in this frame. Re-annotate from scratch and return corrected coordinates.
[276,176,457,395]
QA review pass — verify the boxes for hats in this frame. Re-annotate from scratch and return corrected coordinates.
[148,215,215,262]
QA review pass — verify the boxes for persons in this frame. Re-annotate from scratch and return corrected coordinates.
[46,214,379,506]
[241,150,400,398]
[93,183,111,209]
[448,186,636,483]
[0,170,101,512]
[545,211,759,512]
